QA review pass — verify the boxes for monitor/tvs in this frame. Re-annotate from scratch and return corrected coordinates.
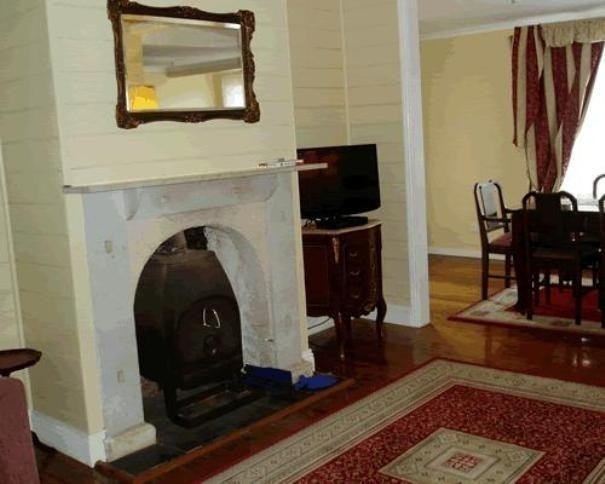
[296,144,381,230]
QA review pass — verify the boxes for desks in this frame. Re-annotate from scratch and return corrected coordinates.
[0,346,58,456]
[503,194,605,314]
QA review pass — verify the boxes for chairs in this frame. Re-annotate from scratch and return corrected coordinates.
[592,172,605,197]
[516,187,588,330]
[468,176,553,303]
[598,193,605,330]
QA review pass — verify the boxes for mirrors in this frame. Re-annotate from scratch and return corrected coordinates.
[107,0,260,127]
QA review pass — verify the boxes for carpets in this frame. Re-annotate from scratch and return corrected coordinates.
[169,356,605,482]
[447,280,605,343]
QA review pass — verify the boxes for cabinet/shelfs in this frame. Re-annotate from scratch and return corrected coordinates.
[302,218,388,363]
